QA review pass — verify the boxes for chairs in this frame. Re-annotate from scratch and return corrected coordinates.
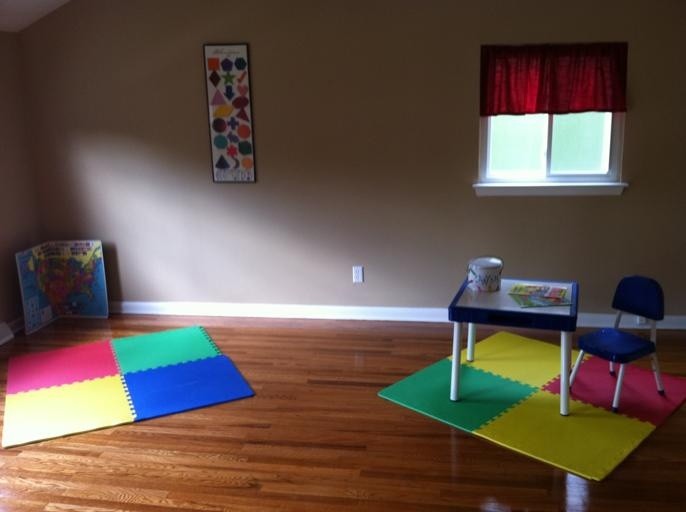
[569,275,664,413]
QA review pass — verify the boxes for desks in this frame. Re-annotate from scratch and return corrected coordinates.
[448,275,578,416]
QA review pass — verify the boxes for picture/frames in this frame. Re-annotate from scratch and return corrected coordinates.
[203,43,256,184]
